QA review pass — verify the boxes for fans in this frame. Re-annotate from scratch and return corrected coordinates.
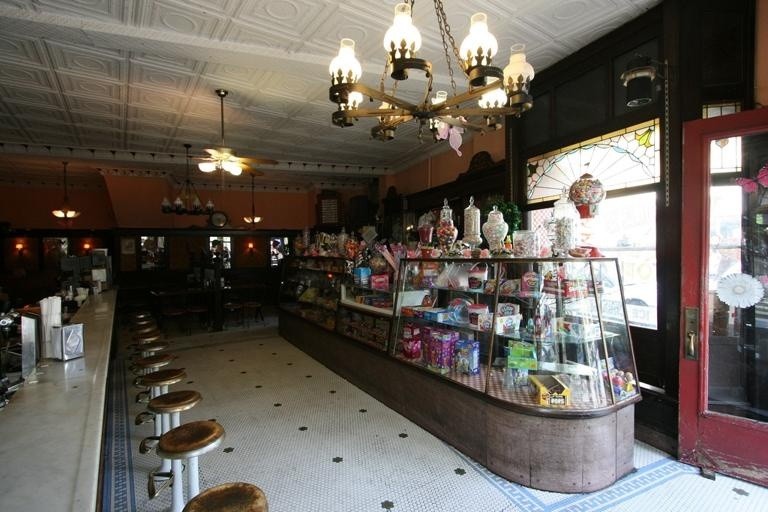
[184,89,278,178]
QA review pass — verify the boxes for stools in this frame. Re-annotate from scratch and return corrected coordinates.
[159,306,187,336]
[158,420,226,512]
[187,303,210,334]
[221,302,244,329]
[126,309,188,435]
[243,301,265,322]
[183,480,266,512]
[146,390,201,480]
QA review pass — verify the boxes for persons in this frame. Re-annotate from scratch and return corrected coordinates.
[271,241,284,261]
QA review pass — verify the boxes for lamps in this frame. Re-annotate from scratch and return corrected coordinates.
[198,92,246,176]
[240,173,264,225]
[49,161,82,220]
[328,0,537,145]
[160,142,219,216]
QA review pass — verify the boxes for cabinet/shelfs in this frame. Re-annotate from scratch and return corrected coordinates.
[275,248,646,482]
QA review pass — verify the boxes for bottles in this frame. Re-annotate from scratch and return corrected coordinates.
[417,184,582,257]
[315,227,359,260]
[295,281,304,298]
[369,253,387,275]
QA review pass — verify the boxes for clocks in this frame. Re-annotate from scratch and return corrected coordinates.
[208,211,228,228]
